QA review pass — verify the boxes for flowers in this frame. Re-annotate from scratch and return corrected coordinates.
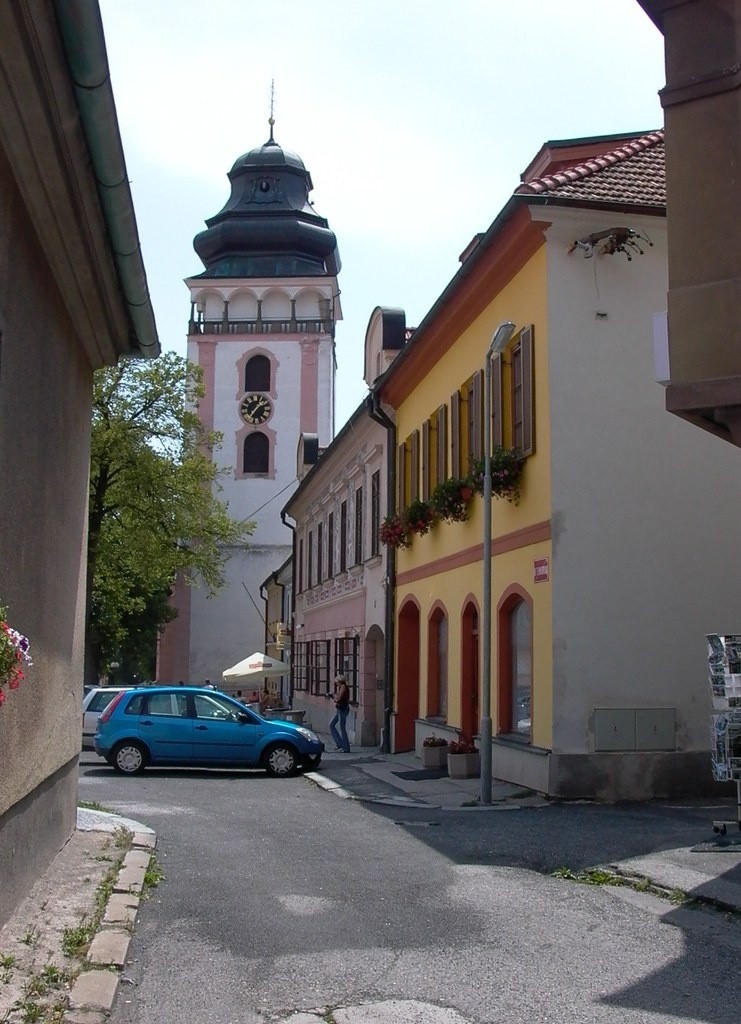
[434,475,473,523]
[423,731,448,747]
[447,729,479,755]
[377,511,408,549]
[470,441,520,510]
[402,490,433,538]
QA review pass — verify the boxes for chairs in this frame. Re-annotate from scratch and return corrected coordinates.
[180,698,189,716]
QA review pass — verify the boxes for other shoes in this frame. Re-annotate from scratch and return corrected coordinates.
[334,745,343,751]
[339,747,350,752]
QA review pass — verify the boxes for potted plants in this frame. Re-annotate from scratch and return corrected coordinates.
[446,752,480,780]
[422,746,449,770]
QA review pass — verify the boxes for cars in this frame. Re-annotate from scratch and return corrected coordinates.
[93,686,326,778]
[82,685,240,752]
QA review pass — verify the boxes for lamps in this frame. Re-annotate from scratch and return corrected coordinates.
[296,623,304,631]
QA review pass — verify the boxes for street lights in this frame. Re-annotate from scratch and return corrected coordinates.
[480,322,517,802]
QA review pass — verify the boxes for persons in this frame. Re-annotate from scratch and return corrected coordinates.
[236,690,247,702]
[249,691,257,700]
[329,674,351,752]
[204,680,213,689]
[262,687,294,710]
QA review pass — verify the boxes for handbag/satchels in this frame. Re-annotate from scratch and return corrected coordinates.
[335,701,345,713]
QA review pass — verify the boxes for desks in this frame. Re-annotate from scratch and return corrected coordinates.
[265,708,288,720]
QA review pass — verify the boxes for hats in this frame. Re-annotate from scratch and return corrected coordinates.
[334,674,346,682]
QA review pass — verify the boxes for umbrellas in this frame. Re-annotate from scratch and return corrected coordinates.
[223,651,290,702]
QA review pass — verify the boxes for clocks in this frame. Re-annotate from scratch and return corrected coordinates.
[238,393,271,425]
